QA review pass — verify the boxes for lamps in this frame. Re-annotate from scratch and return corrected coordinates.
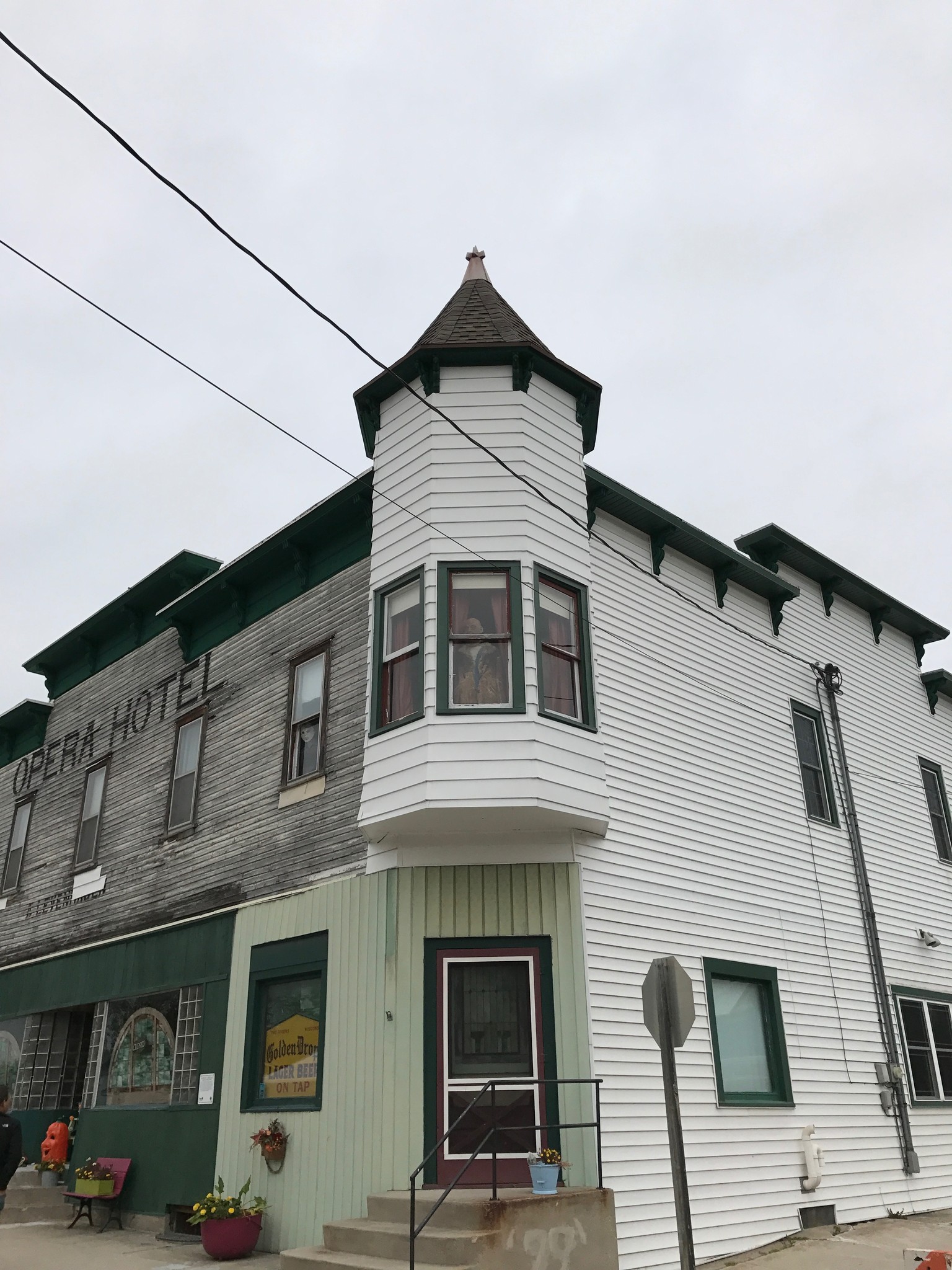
[915,928,941,947]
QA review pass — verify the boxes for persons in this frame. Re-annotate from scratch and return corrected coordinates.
[0,1084,23,1211]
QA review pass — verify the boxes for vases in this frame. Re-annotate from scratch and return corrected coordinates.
[75,1179,114,1195]
[528,1161,560,1195]
[42,1171,59,1187]
[264,1146,285,1161]
[200,1209,263,1258]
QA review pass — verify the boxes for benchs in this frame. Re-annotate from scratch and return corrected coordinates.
[60,1157,132,1233]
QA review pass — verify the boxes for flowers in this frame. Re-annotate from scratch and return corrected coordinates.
[249,1118,290,1151]
[34,1158,66,1175]
[527,1145,571,1170]
[75,1157,116,1180]
[187,1174,272,1226]
